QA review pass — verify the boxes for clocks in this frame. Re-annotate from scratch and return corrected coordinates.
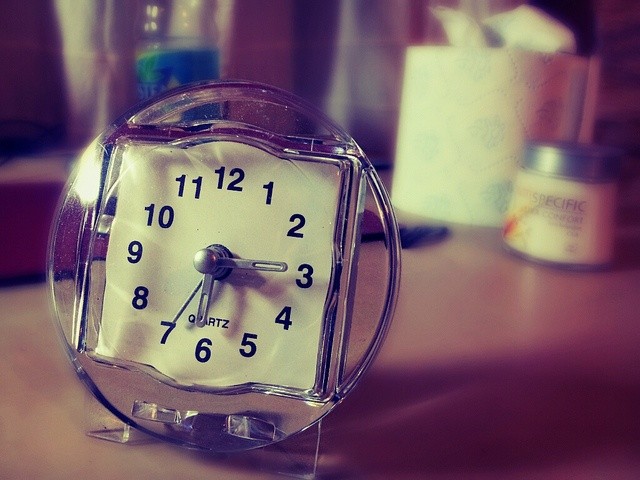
[46,79,402,479]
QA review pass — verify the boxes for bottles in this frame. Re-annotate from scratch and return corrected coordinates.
[131,0,223,120]
[498,136,625,274]
